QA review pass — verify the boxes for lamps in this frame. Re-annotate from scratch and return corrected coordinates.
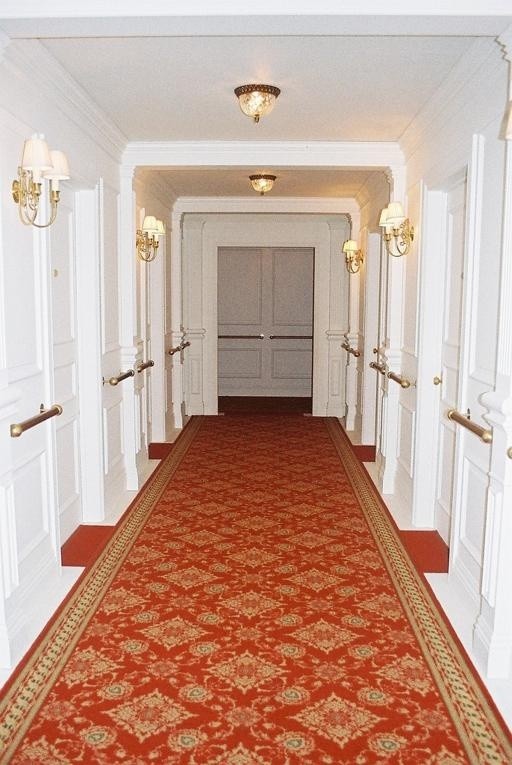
[135,215,166,263]
[11,137,71,227]
[234,83,281,123]
[342,239,365,274]
[249,175,277,198]
[378,202,415,258]
